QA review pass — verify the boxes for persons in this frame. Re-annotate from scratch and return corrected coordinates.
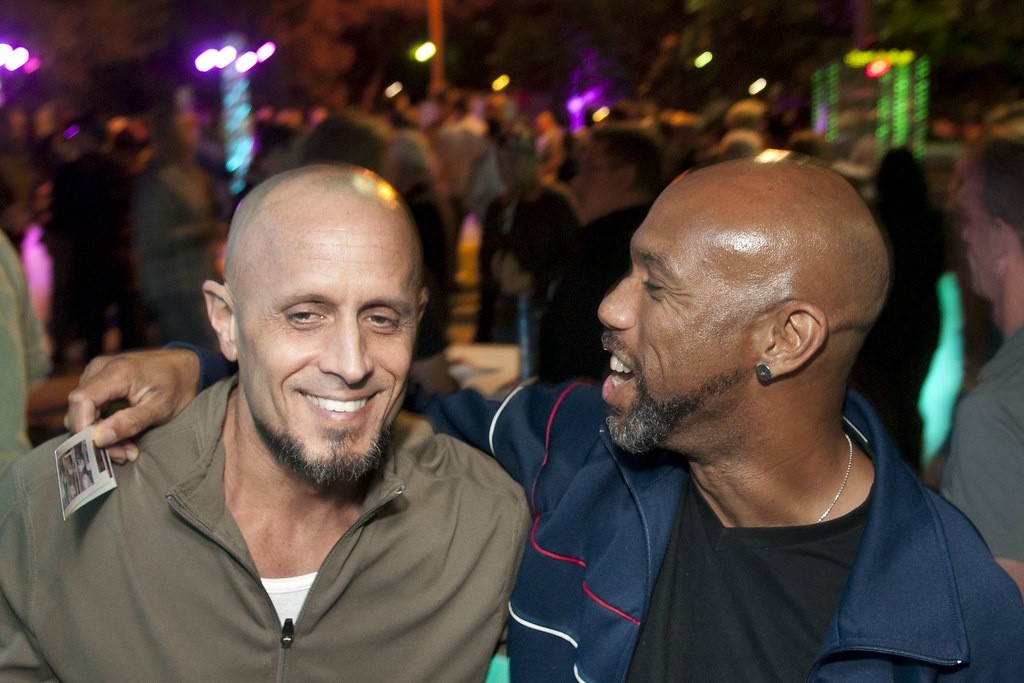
[3,87,827,459]
[939,135,1023,598]
[2,163,532,682]
[850,146,951,477]
[60,158,1024,683]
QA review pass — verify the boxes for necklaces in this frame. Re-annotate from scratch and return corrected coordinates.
[814,429,854,525]
